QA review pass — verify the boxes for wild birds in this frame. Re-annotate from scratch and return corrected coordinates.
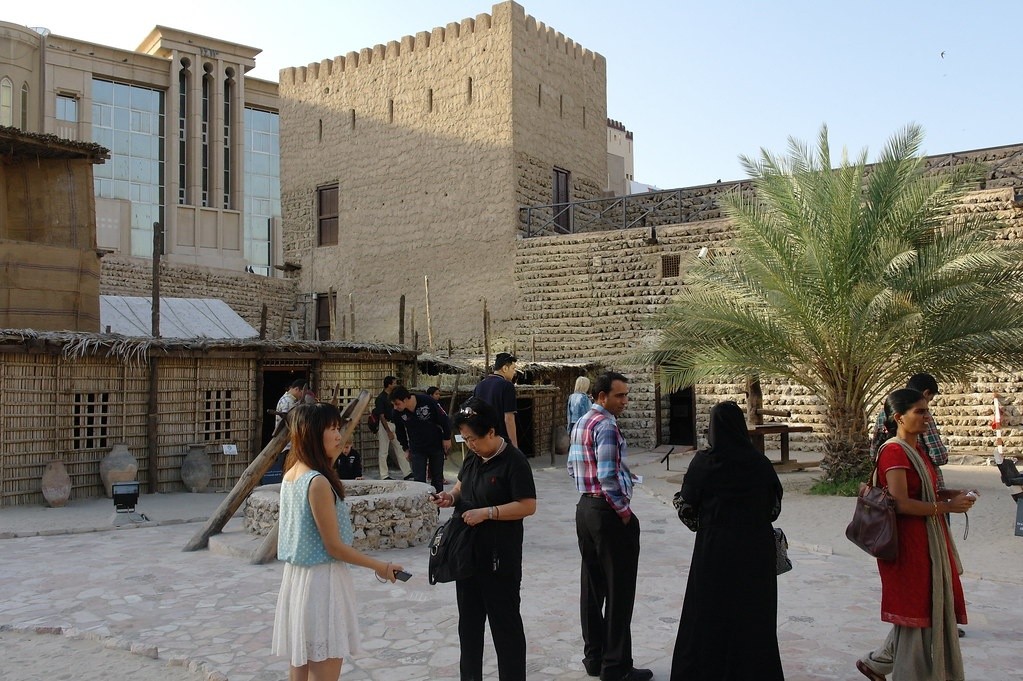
[248,266,254,273]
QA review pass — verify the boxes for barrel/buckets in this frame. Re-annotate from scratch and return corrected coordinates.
[261,454,285,484]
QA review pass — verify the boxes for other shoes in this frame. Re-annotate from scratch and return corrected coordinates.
[403,472,412,480]
[855,659,886,681]
[624,668,654,681]
[382,476,396,480]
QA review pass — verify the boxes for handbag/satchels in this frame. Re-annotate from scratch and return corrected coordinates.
[368,409,378,435]
[846,443,907,561]
[428,510,478,586]
[773,527,793,575]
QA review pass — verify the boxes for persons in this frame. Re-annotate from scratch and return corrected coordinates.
[474,353,519,449]
[375,376,417,480]
[429,396,537,681]
[272,379,308,470]
[567,376,593,451]
[670,401,784,681]
[567,371,654,681]
[856,373,981,681]
[389,386,452,494]
[332,440,363,480]
[271,402,403,681]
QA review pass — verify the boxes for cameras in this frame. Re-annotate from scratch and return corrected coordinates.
[393,570,412,582]
[966,491,978,498]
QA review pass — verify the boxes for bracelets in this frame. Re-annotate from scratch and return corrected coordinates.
[495,506,499,520]
[488,507,492,519]
[448,493,454,505]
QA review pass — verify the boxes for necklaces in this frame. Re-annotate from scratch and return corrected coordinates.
[482,437,504,459]
[494,374,504,378]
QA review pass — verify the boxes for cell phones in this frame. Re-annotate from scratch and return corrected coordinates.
[427,491,439,500]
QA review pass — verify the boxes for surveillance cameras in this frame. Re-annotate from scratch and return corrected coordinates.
[697,247,709,260]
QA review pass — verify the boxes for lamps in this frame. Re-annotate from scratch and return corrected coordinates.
[111,481,139,512]
